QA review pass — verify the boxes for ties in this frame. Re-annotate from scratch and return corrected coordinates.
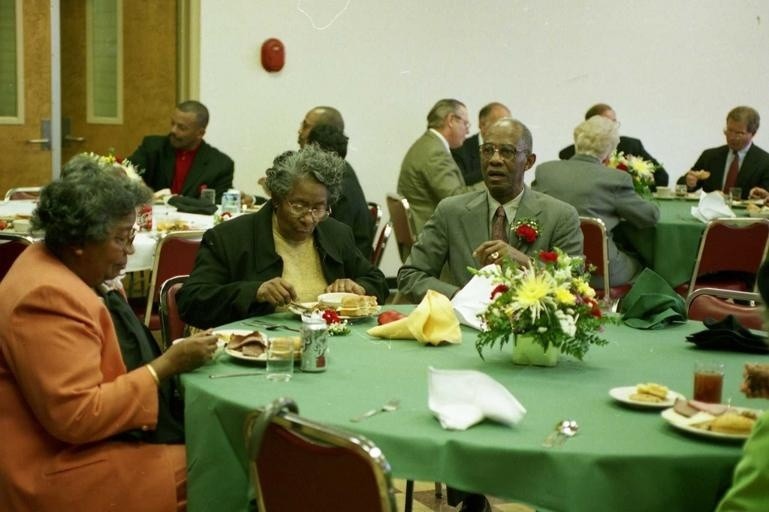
[491,207,509,243]
[724,150,739,194]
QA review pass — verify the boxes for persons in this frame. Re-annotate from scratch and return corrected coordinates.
[677,105,769,200]
[174,139,389,342]
[396,116,585,510]
[559,103,669,192]
[127,99,234,206]
[397,96,512,263]
[1,149,221,511]
[257,106,375,262]
[536,115,660,290]
[712,253,769,511]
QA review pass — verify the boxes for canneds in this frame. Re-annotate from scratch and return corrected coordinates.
[301,319,327,373]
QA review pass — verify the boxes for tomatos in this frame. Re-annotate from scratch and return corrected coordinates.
[324,311,339,325]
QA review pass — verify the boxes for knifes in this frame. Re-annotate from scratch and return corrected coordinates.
[209,370,267,378]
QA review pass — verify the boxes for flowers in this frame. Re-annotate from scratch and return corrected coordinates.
[606,149,663,199]
[512,217,540,245]
[468,248,627,362]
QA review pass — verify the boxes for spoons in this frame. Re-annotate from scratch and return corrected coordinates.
[241,322,280,330]
[543,419,579,450]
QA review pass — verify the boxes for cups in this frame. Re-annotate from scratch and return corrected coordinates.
[694,359,726,404]
[265,337,294,382]
[221,193,241,213]
[201,189,215,204]
[654,185,742,202]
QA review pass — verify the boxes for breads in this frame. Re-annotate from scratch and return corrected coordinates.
[631,392,662,405]
[674,396,757,435]
[267,338,303,360]
[637,385,669,400]
[341,296,383,319]
[689,168,709,182]
[226,329,265,356]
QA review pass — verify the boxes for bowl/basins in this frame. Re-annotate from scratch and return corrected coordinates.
[318,293,359,304]
[12,219,31,232]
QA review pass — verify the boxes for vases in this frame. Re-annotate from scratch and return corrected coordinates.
[514,332,559,370]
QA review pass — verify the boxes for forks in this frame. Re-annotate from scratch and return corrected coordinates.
[350,398,403,423]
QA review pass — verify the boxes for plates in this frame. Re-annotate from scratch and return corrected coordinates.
[224,346,299,364]
[288,302,373,322]
[608,386,686,409]
[660,405,764,440]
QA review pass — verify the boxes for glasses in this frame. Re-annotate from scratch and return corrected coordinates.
[476,144,532,163]
[116,229,136,248]
[284,195,333,222]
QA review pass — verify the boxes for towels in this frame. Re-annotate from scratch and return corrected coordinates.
[425,365,527,432]
[690,186,736,225]
[367,289,462,346]
[690,315,765,353]
[618,266,688,330]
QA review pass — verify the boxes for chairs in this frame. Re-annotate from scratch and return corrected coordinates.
[128,230,202,333]
[0,231,31,284]
[674,219,768,296]
[242,408,399,512]
[578,217,632,301]
[687,287,769,332]
[159,275,194,347]
[371,219,391,267]
[385,192,417,261]
[5,186,44,201]
[367,202,379,225]
